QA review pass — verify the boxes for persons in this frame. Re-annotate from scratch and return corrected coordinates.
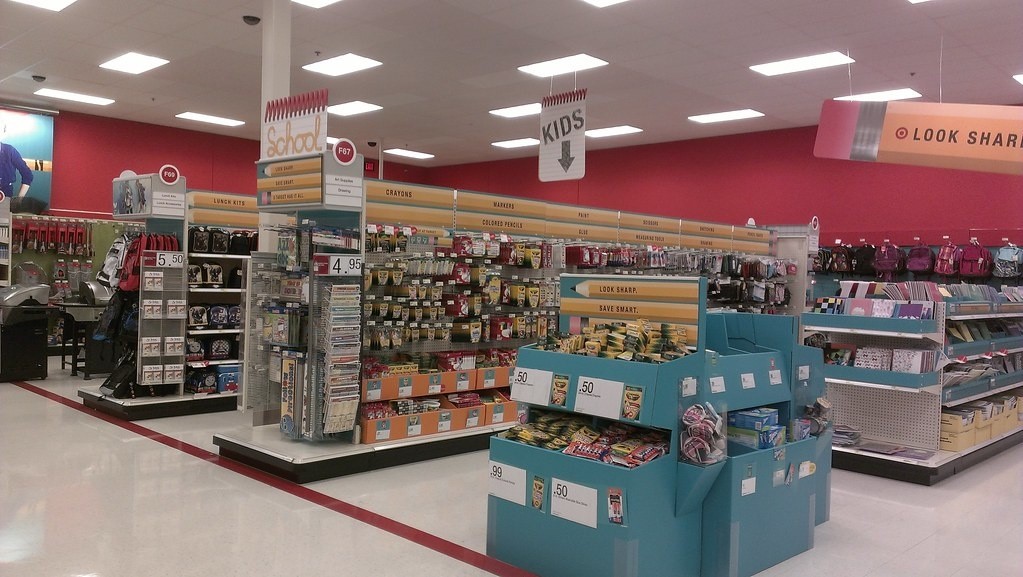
[0,117,33,199]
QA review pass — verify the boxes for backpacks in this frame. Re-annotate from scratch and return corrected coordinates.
[906,240,934,273]
[829,244,854,274]
[934,240,960,276]
[855,244,876,276]
[872,241,904,274]
[90,230,179,399]
[812,246,831,274]
[959,240,992,278]
[992,242,1022,279]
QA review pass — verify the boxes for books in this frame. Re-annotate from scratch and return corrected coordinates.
[821,341,937,374]
[942,386,1023,426]
[942,318,1023,346]
[940,351,1023,390]
[802,278,1023,320]
[828,422,938,465]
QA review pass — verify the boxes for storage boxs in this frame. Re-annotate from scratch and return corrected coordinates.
[726,405,786,449]
[360,346,517,445]
[940,387,1023,452]
[188,365,239,396]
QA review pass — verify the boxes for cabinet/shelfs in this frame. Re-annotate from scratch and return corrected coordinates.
[213,88,816,483]
[800,281,1023,485]
[487,275,830,577]
[78,165,255,425]
